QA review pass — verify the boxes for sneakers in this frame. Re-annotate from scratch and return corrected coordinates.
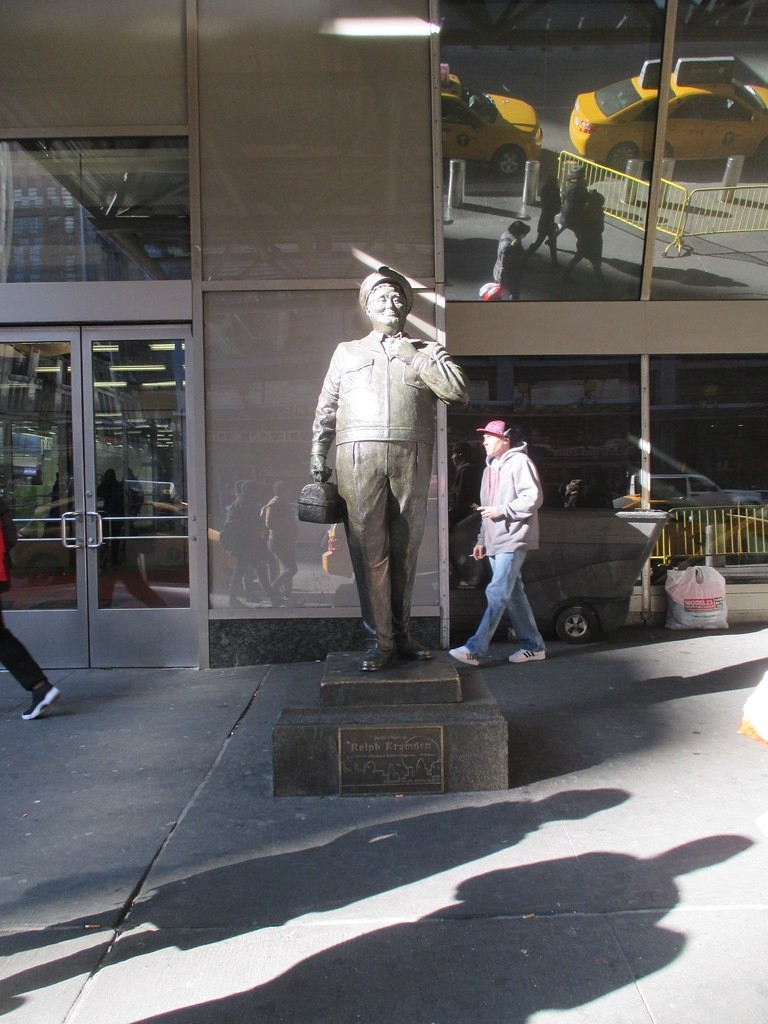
[22,682,60,721]
[509,649,545,662]
[448,646,480,666]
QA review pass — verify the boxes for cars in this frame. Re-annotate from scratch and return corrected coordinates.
[570,56,768,178]
[8,497,192,590]
[627,472,764,521]
[438,63,546,176]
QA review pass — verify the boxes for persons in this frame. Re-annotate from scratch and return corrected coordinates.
[220,479,300,609]
[493,221,531,300]
[448,440,568,603]
[543,164,605,299]
[98,539,170,608]
[564,479,586,508]
[31,470,43,485]
[525,176,559,267]
[305,267,473,670]
[0,497,61,719]
[49,466,145,551]
[448,420,547,667]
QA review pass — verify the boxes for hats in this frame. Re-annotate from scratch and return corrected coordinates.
[475,420,512,439]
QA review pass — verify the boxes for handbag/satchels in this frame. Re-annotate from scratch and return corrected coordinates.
[665,566,730,630]
[478,282,505,300]
[581,231,602,253]
